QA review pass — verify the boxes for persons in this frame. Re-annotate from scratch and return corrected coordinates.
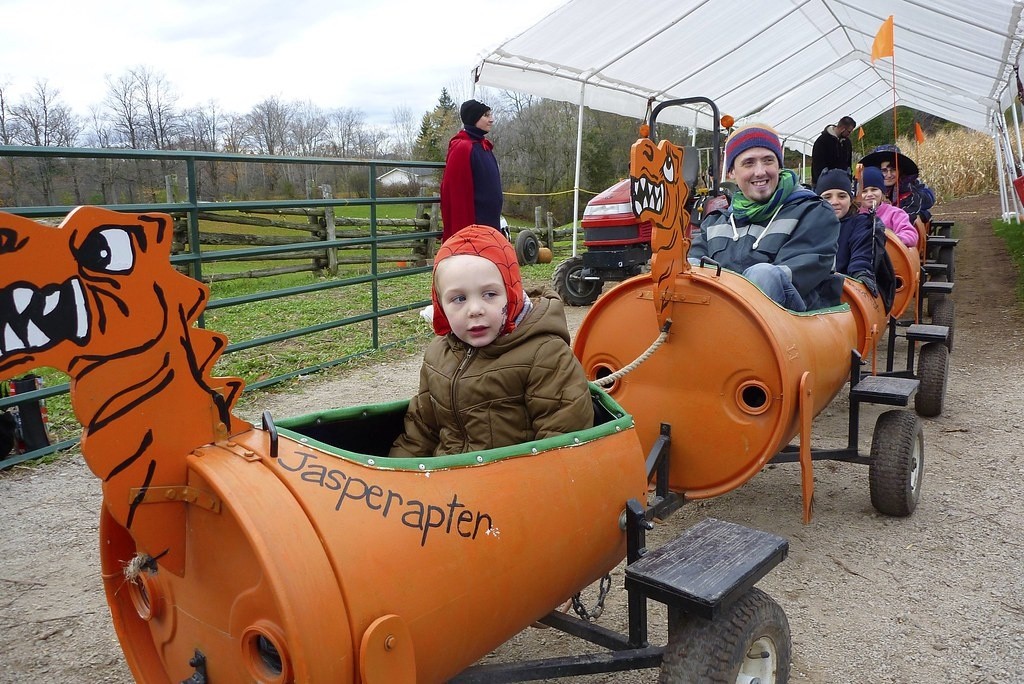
[811,116,856,193]
[440,99,503,235]
[814,167,879,297]
[386,221,595,460]
[686,122,844,311]
[876,158,935,225]
[851,166,919,247]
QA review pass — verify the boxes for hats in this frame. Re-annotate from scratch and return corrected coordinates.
[816,167,853,202]
[433,225,524,336]
[726,124,783,173]
[858,144,918,176]
[855,167,886,195]
[460,100,490,126]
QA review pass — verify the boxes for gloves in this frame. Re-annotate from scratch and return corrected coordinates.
[853,270,878,298]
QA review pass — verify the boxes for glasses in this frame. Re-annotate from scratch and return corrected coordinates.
[880,168,896,174]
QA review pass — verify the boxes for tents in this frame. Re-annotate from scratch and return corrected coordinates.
[462,0,1024,259]
